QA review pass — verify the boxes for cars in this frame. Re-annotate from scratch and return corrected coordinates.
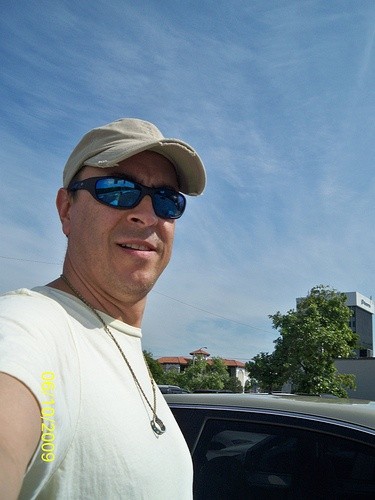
[162,392,375,500]
[157,384,342,399]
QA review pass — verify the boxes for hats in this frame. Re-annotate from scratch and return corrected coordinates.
[64,119,206,196]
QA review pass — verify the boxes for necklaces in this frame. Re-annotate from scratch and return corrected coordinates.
[60,274,166,435]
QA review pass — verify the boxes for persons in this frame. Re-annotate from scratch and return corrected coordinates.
[0,118,207,500]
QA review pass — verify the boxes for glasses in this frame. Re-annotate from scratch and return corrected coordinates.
[69,177,186,218]
